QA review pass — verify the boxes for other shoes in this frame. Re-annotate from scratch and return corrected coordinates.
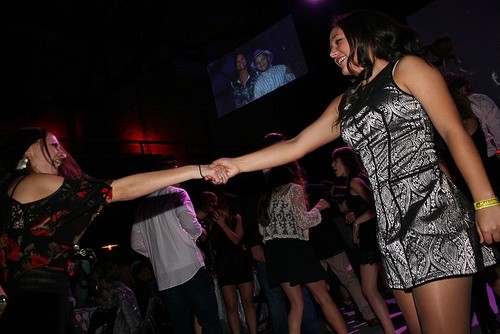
[367,318,383,330]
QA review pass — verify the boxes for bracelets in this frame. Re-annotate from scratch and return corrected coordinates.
[474,196,500,211]
[197,164,206,181]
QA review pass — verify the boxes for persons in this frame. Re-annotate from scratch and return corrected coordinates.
[429,49,500,209]
[198,131,349,334]
[1,126,230,333]
[226,45,298,108]
[205,9,500,334]
[56,232,142,333]
[330,146,399,334]
[130,187,218,334]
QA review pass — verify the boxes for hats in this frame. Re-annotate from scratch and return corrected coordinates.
[250,49,273,70]
[0,126,46,172]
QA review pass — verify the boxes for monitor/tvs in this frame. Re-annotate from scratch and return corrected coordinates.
[207,10,311,119]
